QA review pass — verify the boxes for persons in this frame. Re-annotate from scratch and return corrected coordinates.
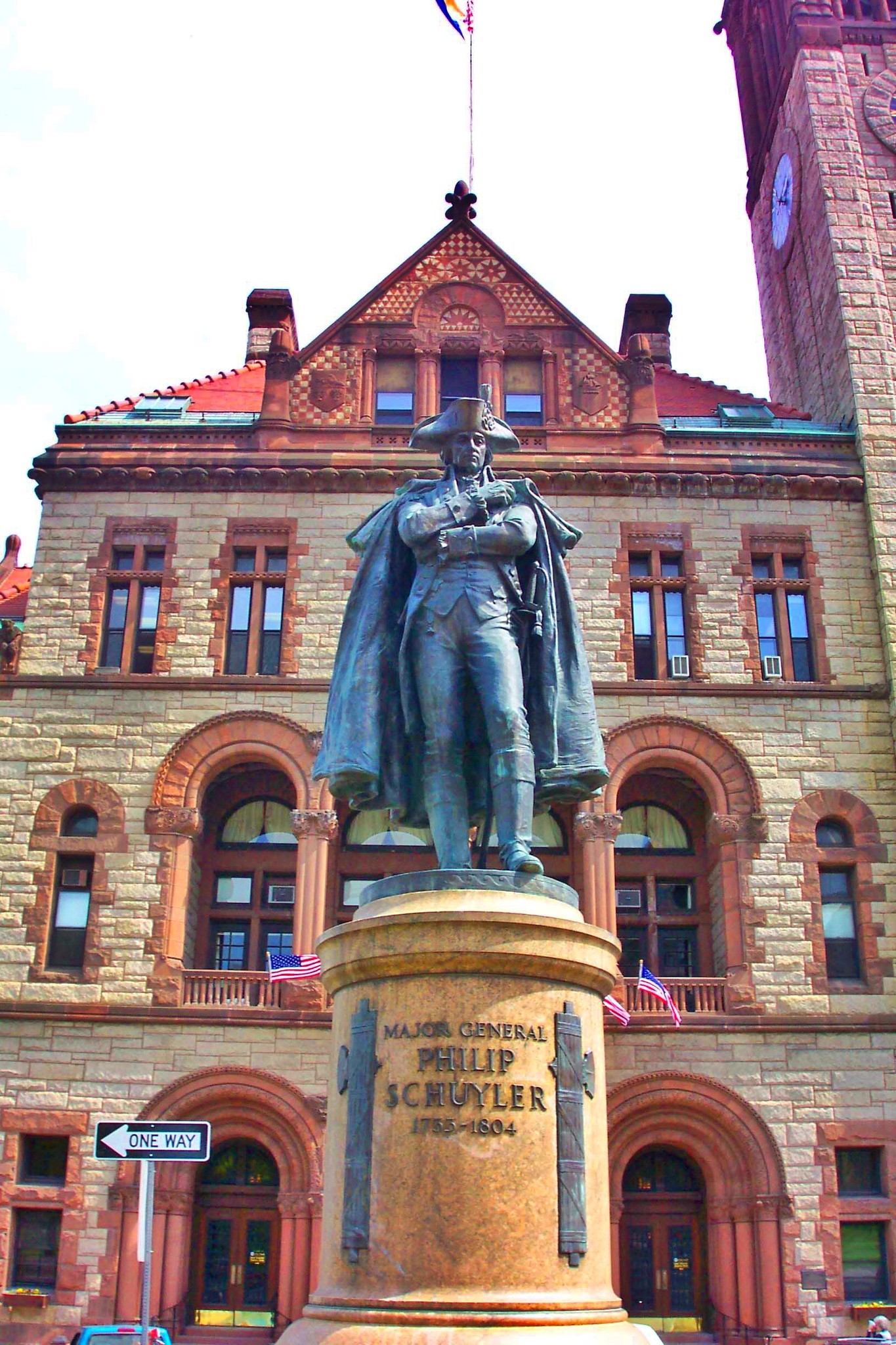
[396,400,543,872]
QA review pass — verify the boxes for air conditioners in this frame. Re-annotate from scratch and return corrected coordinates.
[268,886,295,903]
[670,655,690,678]
[763,655,782,679]
[615,890,641,907]
[61,870,87,886]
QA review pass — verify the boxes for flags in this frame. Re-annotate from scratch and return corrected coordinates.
[637,959,682,1028]
[603,995,631,1026]
[266,952,321,983]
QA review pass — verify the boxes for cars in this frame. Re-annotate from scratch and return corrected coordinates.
[53,1324,172,1345]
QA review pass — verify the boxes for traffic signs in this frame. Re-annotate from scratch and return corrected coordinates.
[94,1120,211,1162]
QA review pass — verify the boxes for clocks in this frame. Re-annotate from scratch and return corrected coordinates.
[770,152,793,249]
